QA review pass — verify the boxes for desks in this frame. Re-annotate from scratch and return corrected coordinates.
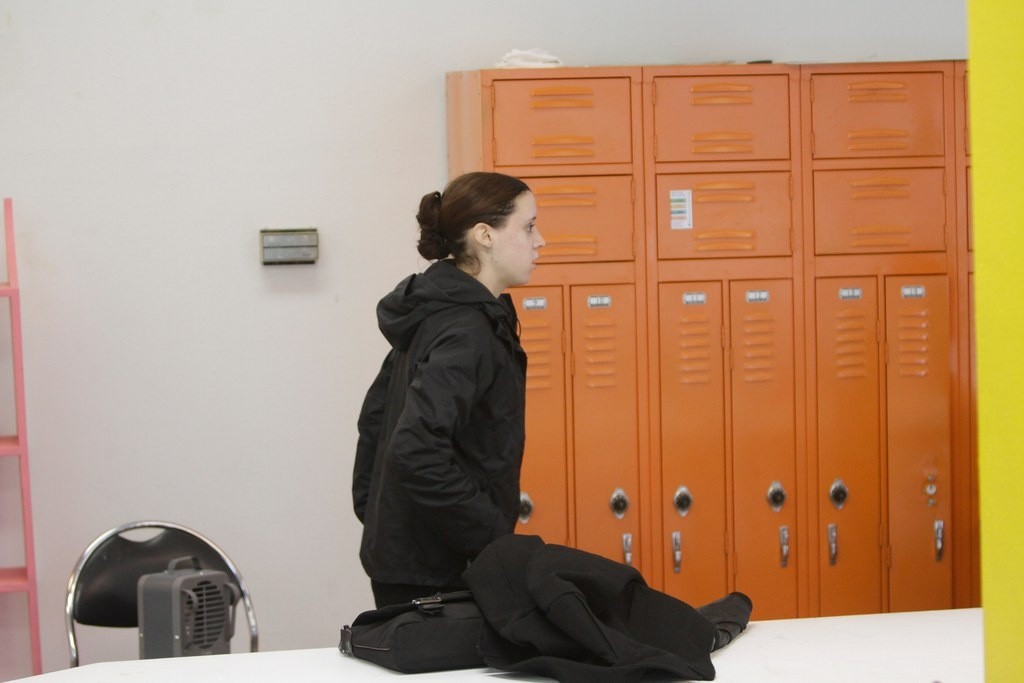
[0,607,983,683]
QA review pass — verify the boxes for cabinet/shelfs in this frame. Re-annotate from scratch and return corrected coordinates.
[0,197,43,675]
[446,60,981,622]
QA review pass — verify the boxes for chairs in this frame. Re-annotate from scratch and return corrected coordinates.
[66,520,259,669]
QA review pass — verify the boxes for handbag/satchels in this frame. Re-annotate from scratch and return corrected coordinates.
[338,590,497,674]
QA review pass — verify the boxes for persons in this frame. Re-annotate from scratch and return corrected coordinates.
[352,172,545,610]
